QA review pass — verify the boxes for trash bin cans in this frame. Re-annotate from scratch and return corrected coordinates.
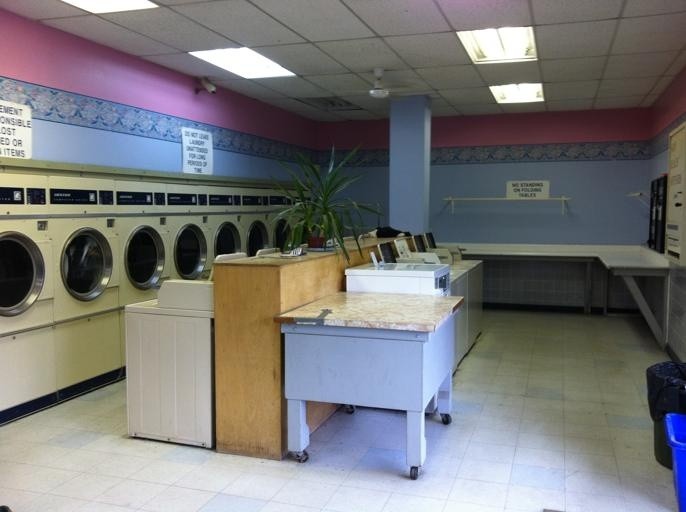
[646,361,686,469]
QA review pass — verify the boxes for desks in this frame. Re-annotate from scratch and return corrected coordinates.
[272,292,464,480]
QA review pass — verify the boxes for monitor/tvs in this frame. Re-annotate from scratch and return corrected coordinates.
[377,242,397,263]
[424,232,437,248]
[412,234,427,252]
[393,237,412,258]
[208,252,248,280]
[255,247,281,256]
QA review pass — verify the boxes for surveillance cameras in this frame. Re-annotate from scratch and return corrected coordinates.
[201,78,217,95]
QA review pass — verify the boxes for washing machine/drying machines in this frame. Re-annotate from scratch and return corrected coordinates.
[345,231,485,381]
[1,156,319,448]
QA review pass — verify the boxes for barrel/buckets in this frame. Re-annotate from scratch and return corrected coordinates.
[664,413,686,512]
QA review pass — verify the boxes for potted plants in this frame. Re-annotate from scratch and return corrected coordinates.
[265,144,387,269]
